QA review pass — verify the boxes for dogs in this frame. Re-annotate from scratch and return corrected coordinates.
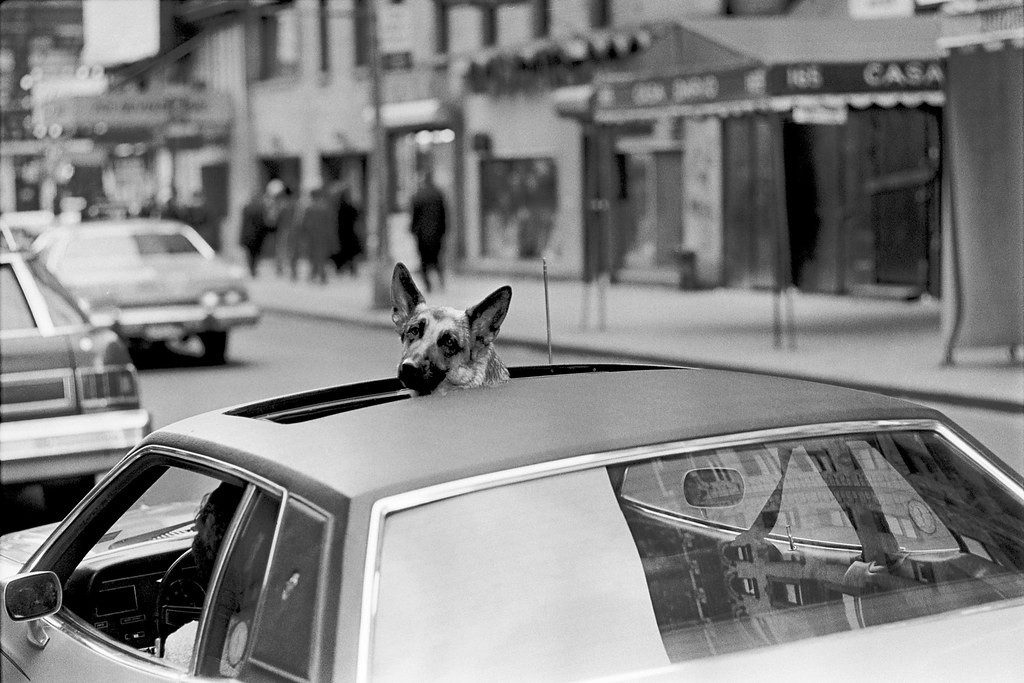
[391,260,513,396]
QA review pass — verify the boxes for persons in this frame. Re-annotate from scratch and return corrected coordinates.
[102,165,450,292]
[140,479,279,677]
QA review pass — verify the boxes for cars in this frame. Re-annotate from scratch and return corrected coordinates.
[31,219,258,363]
[0,360,1024,683]
[0,228,152,521]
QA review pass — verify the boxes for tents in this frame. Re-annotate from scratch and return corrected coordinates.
[568,9,951,352]
